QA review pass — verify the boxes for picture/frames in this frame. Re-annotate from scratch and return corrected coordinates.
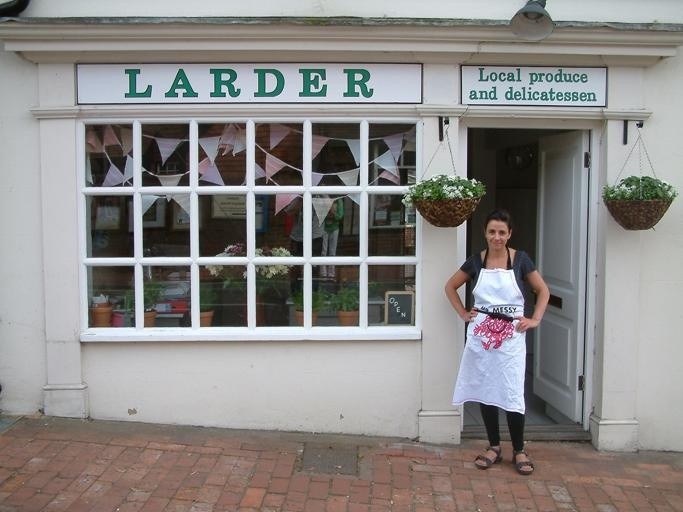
[383,290,418,327]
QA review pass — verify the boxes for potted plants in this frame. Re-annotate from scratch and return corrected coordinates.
[128,276,163,327]
[292,287,325,326]
[330,284,363,327]
[185,284,216,327]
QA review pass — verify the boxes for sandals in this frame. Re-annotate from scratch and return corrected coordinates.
[474,446,502,469]
[512,451,534,475]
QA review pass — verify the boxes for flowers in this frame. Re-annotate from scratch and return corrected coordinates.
[401,172,487,203]
[201,243,294,285]
[599,175,678,203]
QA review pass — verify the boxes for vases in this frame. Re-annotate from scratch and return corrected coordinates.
[243,288,271,325]
[91,303,115,327]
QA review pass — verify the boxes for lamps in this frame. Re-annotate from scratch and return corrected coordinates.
[511,0,551,45]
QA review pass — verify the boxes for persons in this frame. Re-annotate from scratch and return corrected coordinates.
[284,181,334,293]
[445,209,550,474]
[319,197,344,277]
